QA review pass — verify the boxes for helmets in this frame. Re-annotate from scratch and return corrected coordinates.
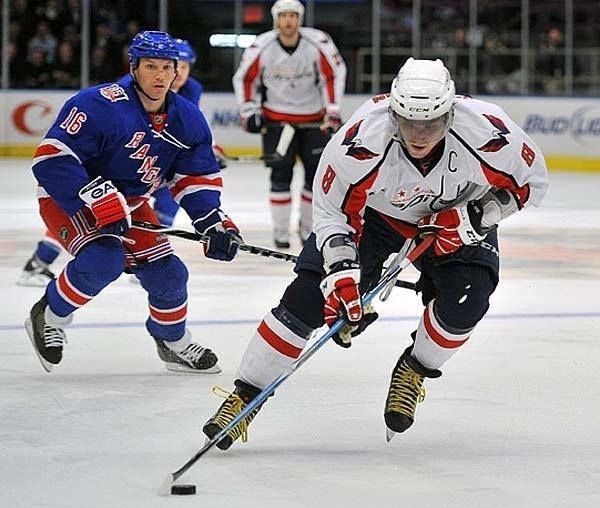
[271,0,305,29]
[128,31,196,70]
[387,57,456,142]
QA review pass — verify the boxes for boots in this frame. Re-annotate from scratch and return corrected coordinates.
[31,278,73,364]
[203,379,274,450]
[155,329,218,369]
[384,330,442,432]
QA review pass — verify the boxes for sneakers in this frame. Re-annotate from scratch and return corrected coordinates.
[274,225,311,247]
[25,254,54,278]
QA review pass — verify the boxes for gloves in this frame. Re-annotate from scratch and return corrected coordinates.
[320,110,341,134]
[78,175,132,234]
[192,207,244,261]
[319,268,378,347]
[417,199,487,259]
[239,101,263,133]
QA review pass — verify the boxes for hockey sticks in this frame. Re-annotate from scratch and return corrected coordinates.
[133,221,428,297]
[222,125,299,169]
[156,238,434,494]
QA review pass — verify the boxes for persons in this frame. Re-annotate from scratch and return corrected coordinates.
[28,28,243,370]
[3,0,600,97]
[20,40,206,288]
[201,54,549,451]
[230,0,346,252]
